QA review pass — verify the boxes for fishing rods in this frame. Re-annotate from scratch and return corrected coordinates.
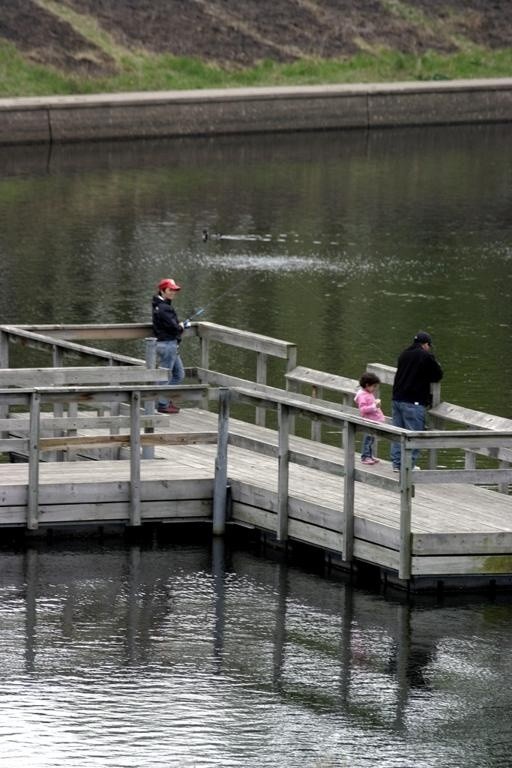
[183,270,262,326]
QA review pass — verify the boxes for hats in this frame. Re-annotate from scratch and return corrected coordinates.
[159,279,180,292]
[413,331,434,347]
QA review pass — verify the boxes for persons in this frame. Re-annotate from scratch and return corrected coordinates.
[355,372,385,465]
[391,331,444,471]
[152,278,191,412]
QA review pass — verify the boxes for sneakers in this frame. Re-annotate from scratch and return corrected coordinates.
[411,464,421,471]
[392,467,401,472]
[361,457,380,466]
[158,404,181,415]
